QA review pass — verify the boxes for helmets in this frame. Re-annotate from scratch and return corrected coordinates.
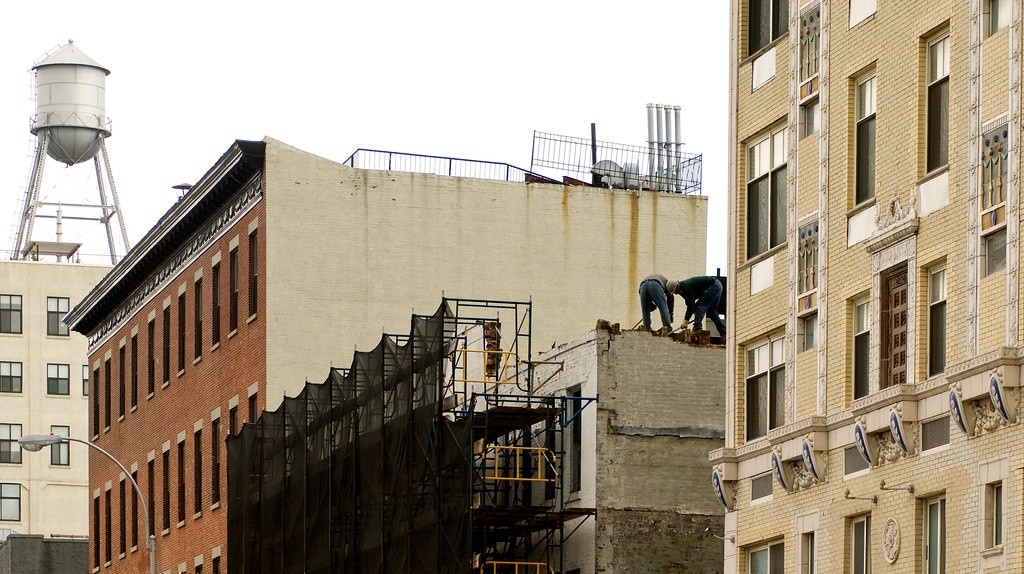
[666,280,678,295]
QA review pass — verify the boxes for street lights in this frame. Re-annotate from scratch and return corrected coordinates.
[17,434,156,574]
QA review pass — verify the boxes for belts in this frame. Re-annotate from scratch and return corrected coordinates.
[644,279,658,282]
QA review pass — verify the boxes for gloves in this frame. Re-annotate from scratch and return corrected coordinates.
[680,319,689,329]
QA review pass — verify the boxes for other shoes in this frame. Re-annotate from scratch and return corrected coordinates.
[663,323,672,331]
[692,325,701,331]
[645,326,652,332]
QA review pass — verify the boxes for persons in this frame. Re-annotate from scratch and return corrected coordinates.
[638,274,674,331]
[666,276,726,336]
